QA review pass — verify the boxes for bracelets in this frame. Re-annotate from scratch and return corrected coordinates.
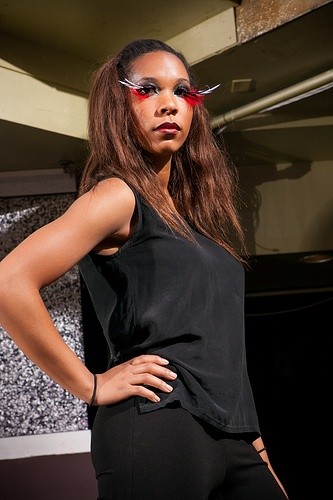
[88,374,100,407]
[256,445,267,454]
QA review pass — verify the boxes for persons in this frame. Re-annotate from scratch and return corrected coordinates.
[0,39,290,499]
